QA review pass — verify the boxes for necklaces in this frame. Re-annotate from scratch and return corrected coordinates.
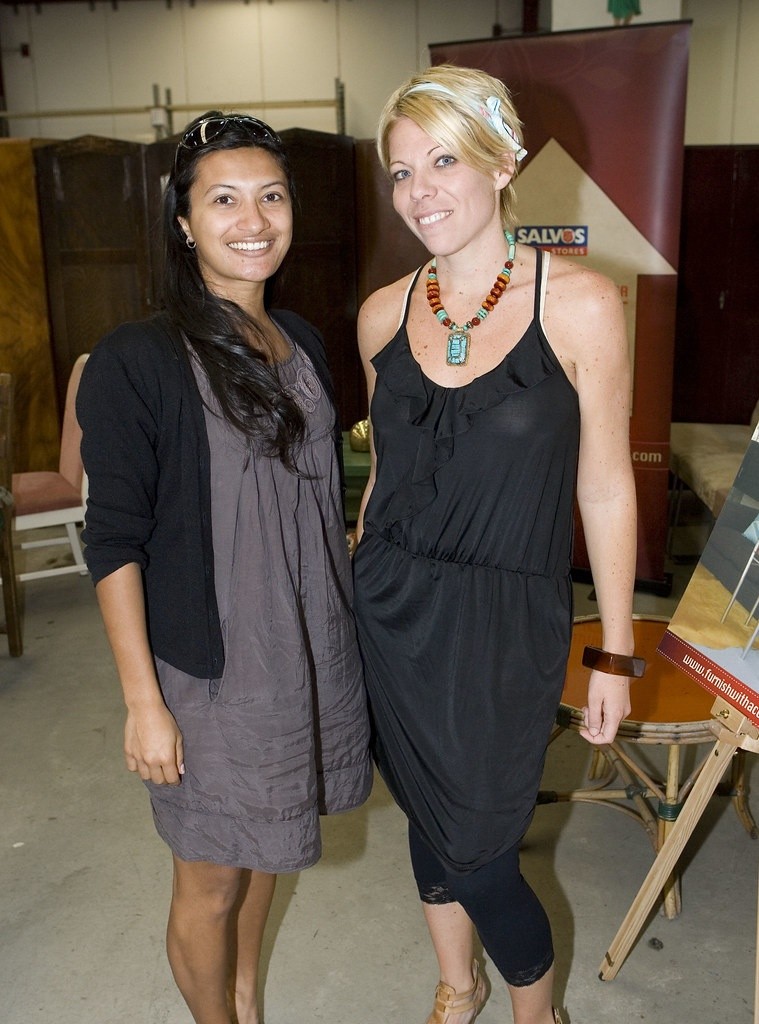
[427,229,515,367]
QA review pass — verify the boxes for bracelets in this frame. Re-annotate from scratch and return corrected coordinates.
[582,645,646,677]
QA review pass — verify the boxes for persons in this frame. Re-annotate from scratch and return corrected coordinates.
[76,110,373,1024]
[350,64,636,1024]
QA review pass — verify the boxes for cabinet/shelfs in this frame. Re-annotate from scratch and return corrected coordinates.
[339,431,373,522]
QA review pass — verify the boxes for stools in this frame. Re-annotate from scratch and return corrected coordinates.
[534,613,759,920]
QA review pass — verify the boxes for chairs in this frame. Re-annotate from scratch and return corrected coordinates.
[1,350,103,587]
[1,371,25,659]
[668,399,759,564]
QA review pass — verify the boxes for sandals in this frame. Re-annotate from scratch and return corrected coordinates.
[422,959,489,1024]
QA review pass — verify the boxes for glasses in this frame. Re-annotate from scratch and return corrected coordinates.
[173,116,286,173]
